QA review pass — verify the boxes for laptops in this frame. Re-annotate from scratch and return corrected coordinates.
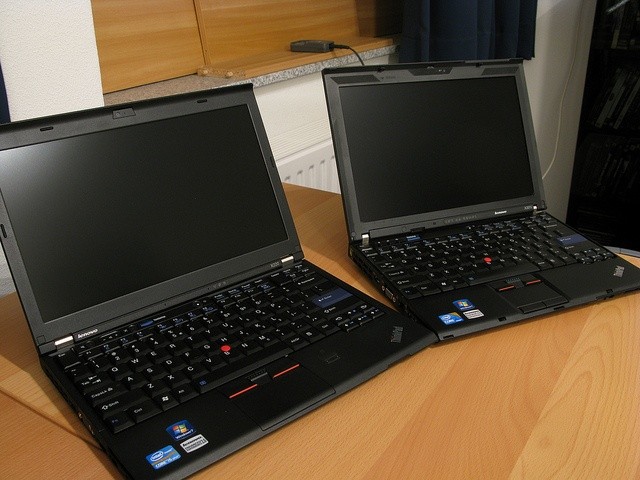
[0,83,438,476]
[321,58,640,342]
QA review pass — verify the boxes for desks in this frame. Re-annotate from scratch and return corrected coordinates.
[0,181,637,480]
